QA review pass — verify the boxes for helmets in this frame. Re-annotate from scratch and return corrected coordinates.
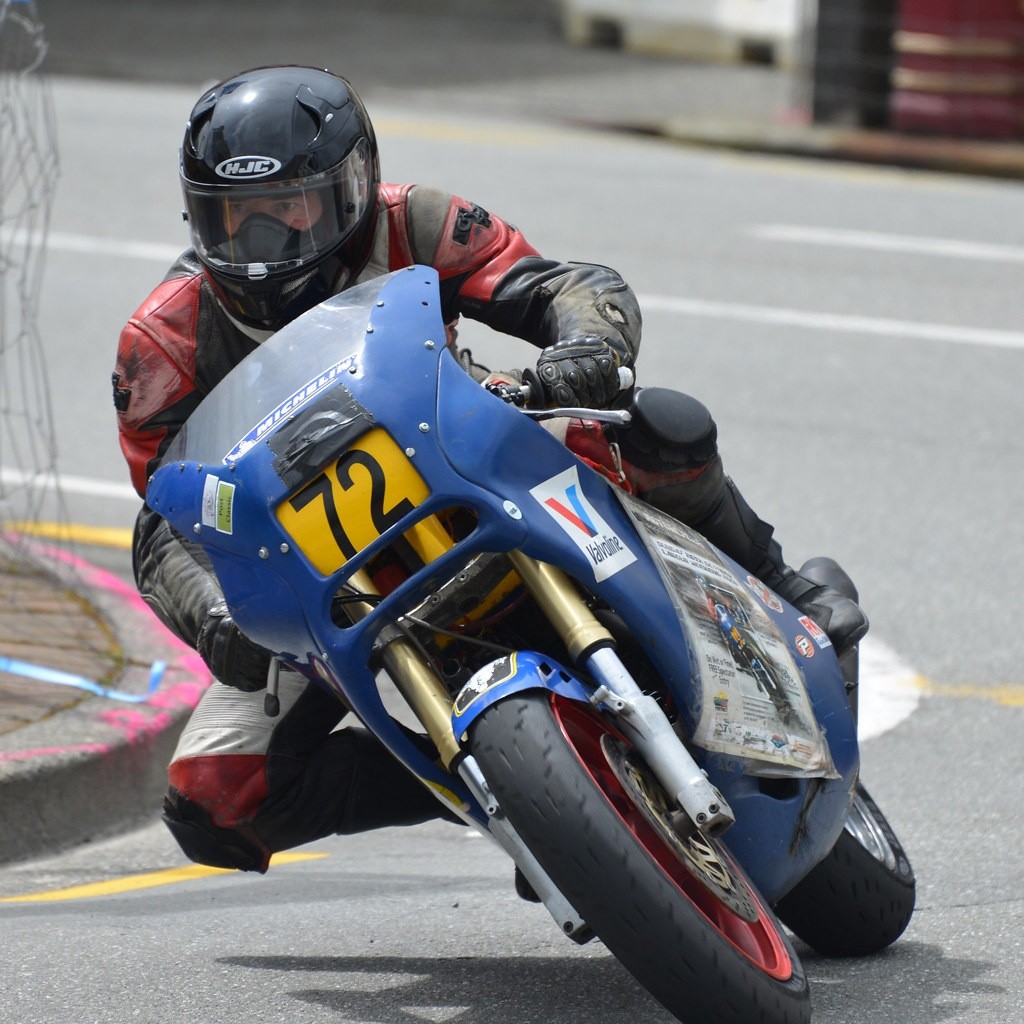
[178,65,379,328]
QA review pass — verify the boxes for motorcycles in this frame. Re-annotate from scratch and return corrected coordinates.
[146,264,915,1024]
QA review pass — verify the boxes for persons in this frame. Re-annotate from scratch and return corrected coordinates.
[112,65,869,875]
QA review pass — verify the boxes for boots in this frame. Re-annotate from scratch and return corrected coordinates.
[651,459,870,659]
[333,724,542,903]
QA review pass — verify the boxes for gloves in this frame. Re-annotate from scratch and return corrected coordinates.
[196,604,269,693]
[536,336,620,407]
